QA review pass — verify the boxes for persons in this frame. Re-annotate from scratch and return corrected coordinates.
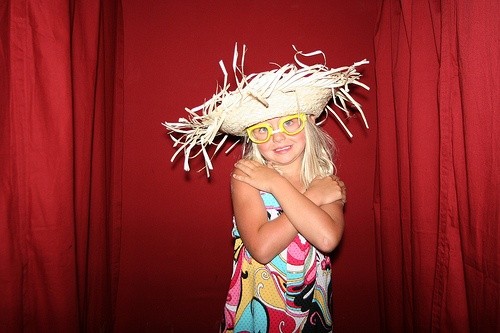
[160,41,370,333]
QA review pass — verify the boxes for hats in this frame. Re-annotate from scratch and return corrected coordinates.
[161,41,369,177]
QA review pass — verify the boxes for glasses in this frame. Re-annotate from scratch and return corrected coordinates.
[246,113,309,144]
